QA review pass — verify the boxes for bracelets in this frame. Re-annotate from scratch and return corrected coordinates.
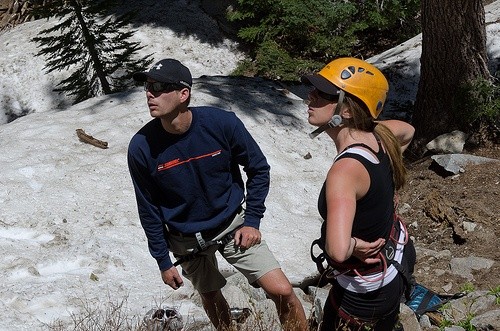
[352,237,357,256]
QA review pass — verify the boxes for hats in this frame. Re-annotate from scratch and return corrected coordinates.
[133,58,192,95]
[300,74,340,95]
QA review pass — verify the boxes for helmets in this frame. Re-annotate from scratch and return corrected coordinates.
[317,57,390,120]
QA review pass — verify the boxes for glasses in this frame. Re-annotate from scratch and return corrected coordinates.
[143,80,183,92]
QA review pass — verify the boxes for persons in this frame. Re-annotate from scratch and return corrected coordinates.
[127,58,308,330]
[306,58,416,331]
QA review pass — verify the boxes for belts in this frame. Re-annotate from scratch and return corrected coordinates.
[162,205,244,238]
